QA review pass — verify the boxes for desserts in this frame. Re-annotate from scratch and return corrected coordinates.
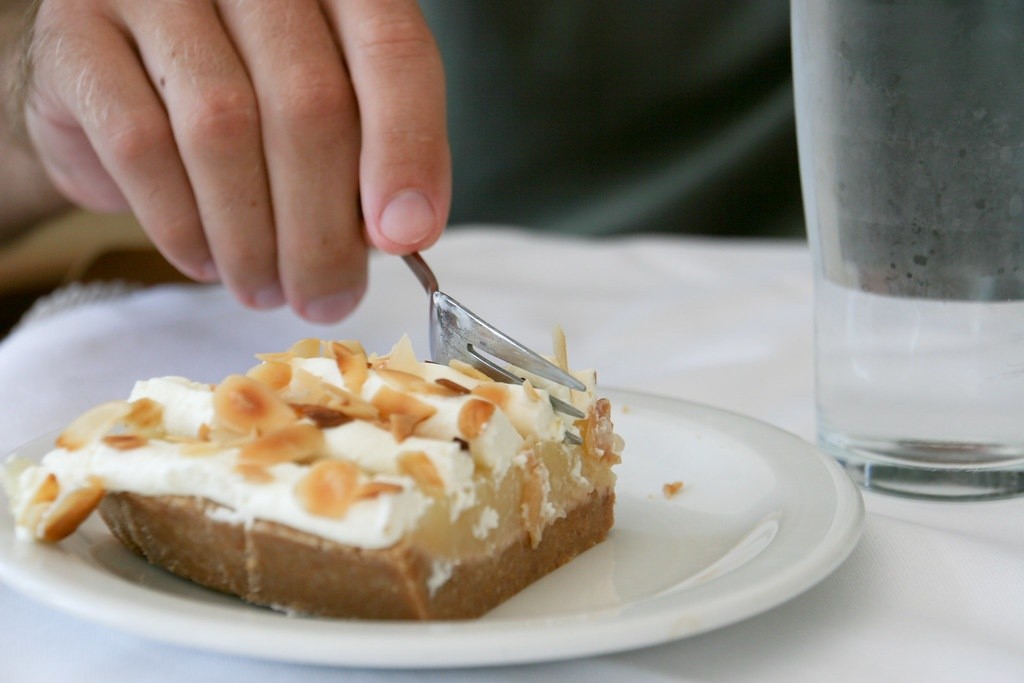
[10,328,629,620]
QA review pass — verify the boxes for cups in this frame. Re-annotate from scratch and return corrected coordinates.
[791,0,1024,497]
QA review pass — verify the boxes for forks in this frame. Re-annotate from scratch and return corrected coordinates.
[401,251,587,445]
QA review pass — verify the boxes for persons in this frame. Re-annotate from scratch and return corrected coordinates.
[0,0,1024,322]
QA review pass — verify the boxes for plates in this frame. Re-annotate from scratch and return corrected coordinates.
[0,379,865,670]
